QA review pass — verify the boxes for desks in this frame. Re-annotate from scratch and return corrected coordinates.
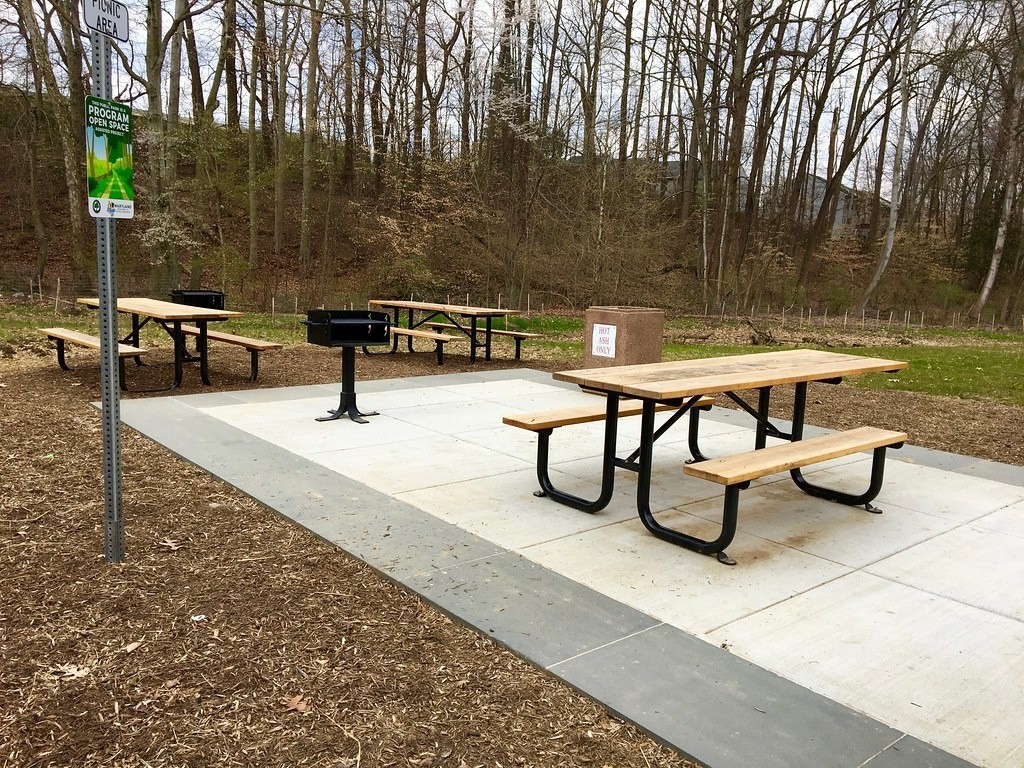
[553,348,908,555]
[76,296,243,393]
[370,300,522,366]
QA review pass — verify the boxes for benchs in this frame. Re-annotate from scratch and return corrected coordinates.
[502,395,715,513]
[423,322,544,363]
[684,426,908,566]
[363,326,466,365]
[38,327,147,394]
[158,324,284,388]
